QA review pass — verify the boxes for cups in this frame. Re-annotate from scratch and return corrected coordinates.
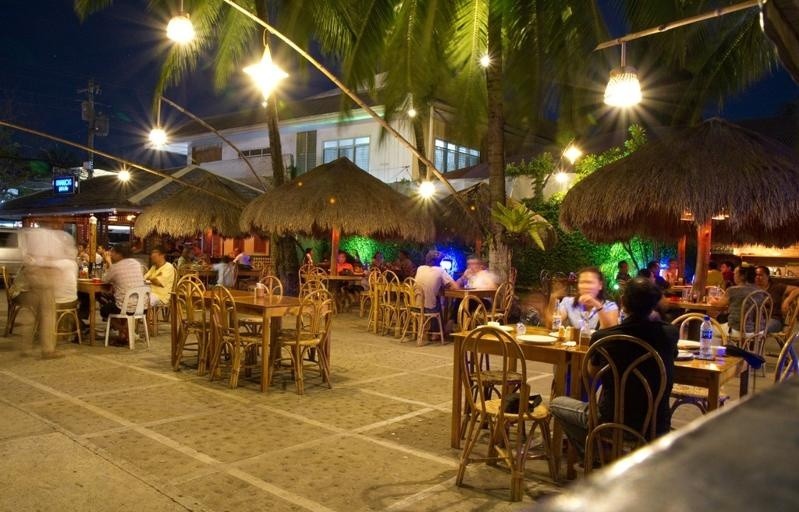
[464,280,474,289]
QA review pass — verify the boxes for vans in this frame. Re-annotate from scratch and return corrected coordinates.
[109,224,134,250]
[0,226,24,287]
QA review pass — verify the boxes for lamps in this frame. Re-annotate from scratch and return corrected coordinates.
[147,94,174,151]
[239,27,290,109]
[164,0,195,46]
[601,39,645,111]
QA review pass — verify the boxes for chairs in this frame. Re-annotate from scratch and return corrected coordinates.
[2,248,172,356]
[359,267,516,349]
[453,281,798,504]
[171,256,337,396]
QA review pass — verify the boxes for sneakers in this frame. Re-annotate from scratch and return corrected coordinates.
[41,351,66,359]
[116,326,139,344]
[572,461,602,472]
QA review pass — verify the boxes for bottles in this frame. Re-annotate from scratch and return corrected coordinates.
[78,259,107,283]
[477,311,729,362]
[553,298,562,328]
[678,286,724,304]
[568,271,576,280]
[769,266,773,276]
[254,281,265,298]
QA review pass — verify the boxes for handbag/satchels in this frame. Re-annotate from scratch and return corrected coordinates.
[503,383,543,415]
